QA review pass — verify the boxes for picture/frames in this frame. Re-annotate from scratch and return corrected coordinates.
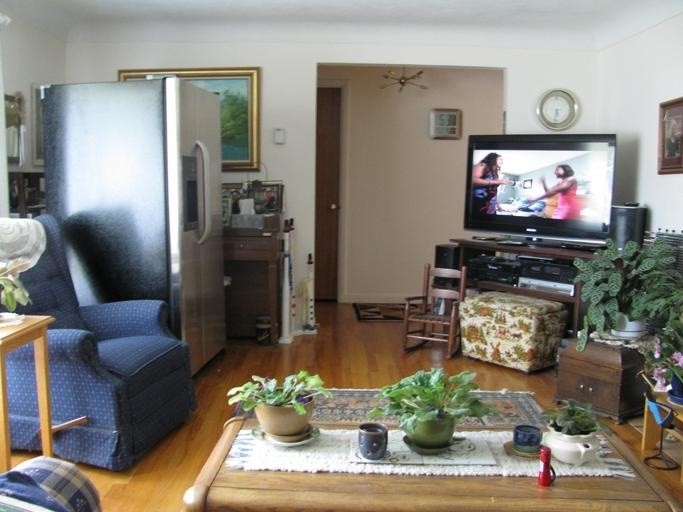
[5,94,23,164]
[31,83,47,166]
[117,67,261,172]
[221,181,283,225]
[656,98,683,175]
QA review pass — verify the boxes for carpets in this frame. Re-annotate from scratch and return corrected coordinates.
[353,302,418,321]
[628,416,683,466]
[232,385,549,434]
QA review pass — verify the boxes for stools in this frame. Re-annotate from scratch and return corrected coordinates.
[458,290,568,373]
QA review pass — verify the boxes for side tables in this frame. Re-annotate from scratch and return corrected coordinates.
[0,312,56,472]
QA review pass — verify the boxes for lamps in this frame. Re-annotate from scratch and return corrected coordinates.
[379,65,429,93]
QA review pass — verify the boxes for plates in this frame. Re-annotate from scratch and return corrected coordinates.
[503,440,542,458]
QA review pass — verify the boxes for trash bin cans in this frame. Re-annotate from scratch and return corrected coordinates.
[256,317,272,346]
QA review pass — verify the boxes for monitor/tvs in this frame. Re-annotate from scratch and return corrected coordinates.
[464,133,616,253]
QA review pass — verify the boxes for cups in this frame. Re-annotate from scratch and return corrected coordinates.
[514,425,541,453]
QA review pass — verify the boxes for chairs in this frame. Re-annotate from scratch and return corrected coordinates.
[0,456,103,512]
[400,262,467,360]
[1,214,197,471]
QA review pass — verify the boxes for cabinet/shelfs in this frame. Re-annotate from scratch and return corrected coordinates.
[8,171,46,218]
[552,339,654,425]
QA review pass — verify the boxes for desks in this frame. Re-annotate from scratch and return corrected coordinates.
[222,227,285,342]
[640,388,683,452]
[449,237,601,339]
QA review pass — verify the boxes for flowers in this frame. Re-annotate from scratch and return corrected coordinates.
[638,327,683,393]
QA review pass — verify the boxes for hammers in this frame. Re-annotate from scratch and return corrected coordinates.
[635,369,655,394]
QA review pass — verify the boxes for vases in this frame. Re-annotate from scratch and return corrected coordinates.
[663,368,683,407]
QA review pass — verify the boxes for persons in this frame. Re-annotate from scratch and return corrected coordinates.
[230,190,241,214]
[522,164,579,221]
[665,113,678,157]
[472,153,512,216]
[264,189,275,210]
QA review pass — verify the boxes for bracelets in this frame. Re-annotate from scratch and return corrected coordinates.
[489,180,491,186]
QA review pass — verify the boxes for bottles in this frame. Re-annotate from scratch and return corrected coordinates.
[538,446,551,486]
[358,422,389,458]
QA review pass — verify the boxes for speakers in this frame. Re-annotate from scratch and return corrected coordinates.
[434,244,458,288]
[610,205,647,254]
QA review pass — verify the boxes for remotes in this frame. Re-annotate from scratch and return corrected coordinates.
[497,239,528,246]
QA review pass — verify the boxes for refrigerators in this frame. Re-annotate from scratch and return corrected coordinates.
[40,77,227,377]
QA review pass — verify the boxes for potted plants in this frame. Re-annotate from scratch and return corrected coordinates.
[365,367,500,449]
[573,235,683,353]
[226,369,333,436]
[543,398,600,466]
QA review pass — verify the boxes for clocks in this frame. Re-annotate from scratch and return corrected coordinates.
[534,87,582,132]
